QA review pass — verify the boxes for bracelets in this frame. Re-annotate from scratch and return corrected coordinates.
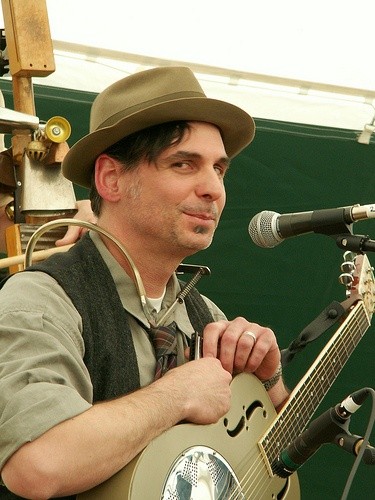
[256,362,284,390]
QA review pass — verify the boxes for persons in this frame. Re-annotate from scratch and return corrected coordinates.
[1,65,299,500]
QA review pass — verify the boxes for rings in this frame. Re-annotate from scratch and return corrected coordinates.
[242,331,257,342]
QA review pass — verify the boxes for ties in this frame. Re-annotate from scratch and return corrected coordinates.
[134,317,177,384]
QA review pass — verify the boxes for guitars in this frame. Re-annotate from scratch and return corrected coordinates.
[93,250,375,500]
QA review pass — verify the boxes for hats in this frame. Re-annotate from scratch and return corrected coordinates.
[61,65,255,189]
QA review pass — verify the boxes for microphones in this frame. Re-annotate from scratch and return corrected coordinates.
[247,204,375,248]
[271,387,370,479]
